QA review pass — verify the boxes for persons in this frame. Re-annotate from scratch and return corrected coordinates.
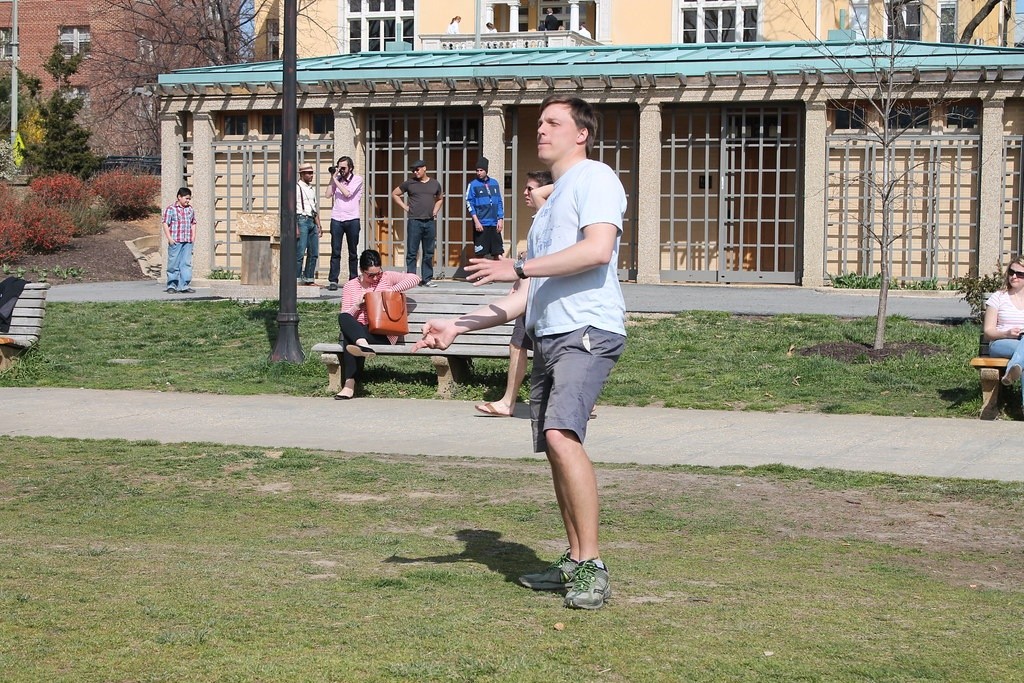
[296,163,322,285]
[335,249,420,399]
[984,256,1024,407]
[410,93,628,610]
[448,16,461,34]
[474,171,597,418]
[391,159,443,287]
[325,156,364,290]
[162,187,196,294]
[580,22,591,37]
[544,8,558,30]
[486,23,497,32]
[465,157,504,284]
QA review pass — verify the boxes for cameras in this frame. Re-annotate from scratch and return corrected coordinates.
[328,166,338,174]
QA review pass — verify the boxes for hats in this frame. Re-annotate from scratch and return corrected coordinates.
[475,157,488,174]
[297,163,314,172]
[409,160,426,168]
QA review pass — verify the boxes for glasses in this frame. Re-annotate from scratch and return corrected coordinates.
[1008,268,1024,279]
[413,167,424,173]
[341,167,350,170]
[363,266,383,279]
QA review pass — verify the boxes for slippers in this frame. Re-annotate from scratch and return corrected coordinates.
[589,414,597,420]
[475,402,513,417]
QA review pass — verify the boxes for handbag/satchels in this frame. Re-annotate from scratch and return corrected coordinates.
[364,291,409,335]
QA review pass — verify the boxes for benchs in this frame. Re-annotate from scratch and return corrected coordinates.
[0,283,51,371]
[970,293,1012,419]
[312,283,536,392]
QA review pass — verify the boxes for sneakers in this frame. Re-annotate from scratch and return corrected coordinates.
[518,549,582,589]
[563,557,611,609]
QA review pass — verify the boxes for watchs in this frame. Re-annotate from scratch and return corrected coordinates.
[513,260,528,279]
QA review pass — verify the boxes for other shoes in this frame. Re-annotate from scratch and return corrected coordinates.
[1001,364,1022,386]
[178,288,195,293]
[329,283,337,291]
[421,281,438,288]
[168,288,176,294]
[334,393,353,400]
[304,280,319,286]
[346,345,377,359]
